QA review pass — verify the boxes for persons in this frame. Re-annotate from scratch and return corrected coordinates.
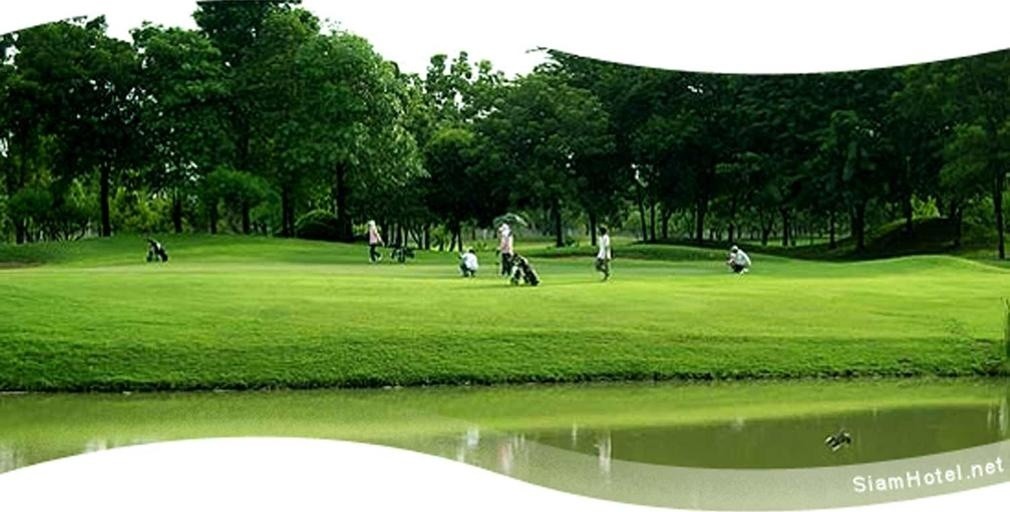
[595,227,611,281]
[369,220,384,263]
[727,245,751,274]
[496,223,514,275]
[459,250,478,277]
